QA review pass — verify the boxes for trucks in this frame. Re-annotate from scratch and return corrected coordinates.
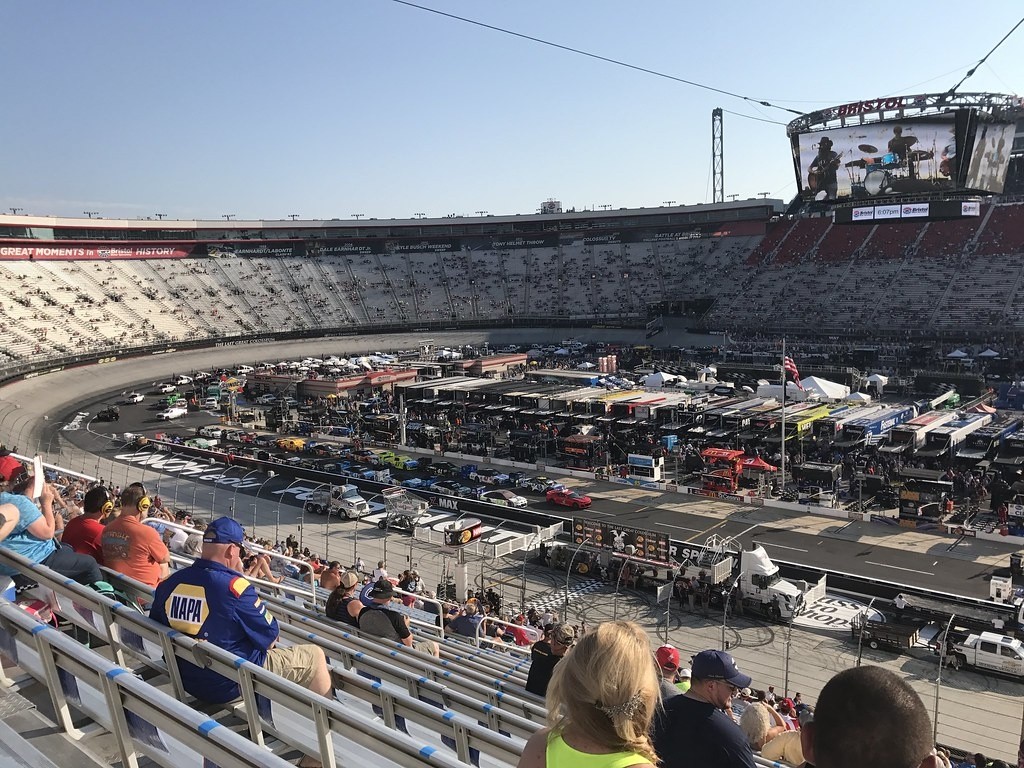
[892,593,1024,632]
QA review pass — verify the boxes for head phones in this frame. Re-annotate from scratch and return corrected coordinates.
[129,482,150,511]
[99,486,113,515]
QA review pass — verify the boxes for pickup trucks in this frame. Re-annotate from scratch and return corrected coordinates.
[935,628,1024,678]
[125,392,146,405]
[156,383,177,394]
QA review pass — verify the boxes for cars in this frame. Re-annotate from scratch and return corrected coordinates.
[479,489,528,509]
[155,345,481,497]
[428,479,471,497]
[498,334,651,359]
[469,467,509,485]
[546,488,592,510]
[520,476,567,494]
[423,460,463,478]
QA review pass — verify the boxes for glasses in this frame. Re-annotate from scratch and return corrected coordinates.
[713,679,743,693]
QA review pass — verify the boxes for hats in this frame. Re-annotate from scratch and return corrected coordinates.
[203,517,252,550]
[369,579,398,599]
[176,510,190,521]
[516,615,525,621]
[741,688,752,696]
[0,456,25,480]
[656,644,680,671]
[692,649,752,689]
[403,596,416,605]
[680,668,691,678]
[547,623,574,647]
[341,570,365,588]
[781,698,795,709]
[797,703,808,716]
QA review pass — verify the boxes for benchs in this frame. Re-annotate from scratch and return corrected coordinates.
[0,517,571,768]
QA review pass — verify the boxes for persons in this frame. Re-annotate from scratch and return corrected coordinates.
[801,666,945,768]
[517,620,664,768]
[808,137,840,200]
[1,446,587,696]
[941,128,956,180]
[655,646,1024,767]
[887,126,914,176]
[647,650,756,768]
[149,517,331,740]
[679,576,710,613]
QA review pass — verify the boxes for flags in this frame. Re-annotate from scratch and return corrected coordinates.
[784,344,802,389]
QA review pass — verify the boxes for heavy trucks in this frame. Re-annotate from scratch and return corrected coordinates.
[545,516,806,622]
[305,478,371,522]
